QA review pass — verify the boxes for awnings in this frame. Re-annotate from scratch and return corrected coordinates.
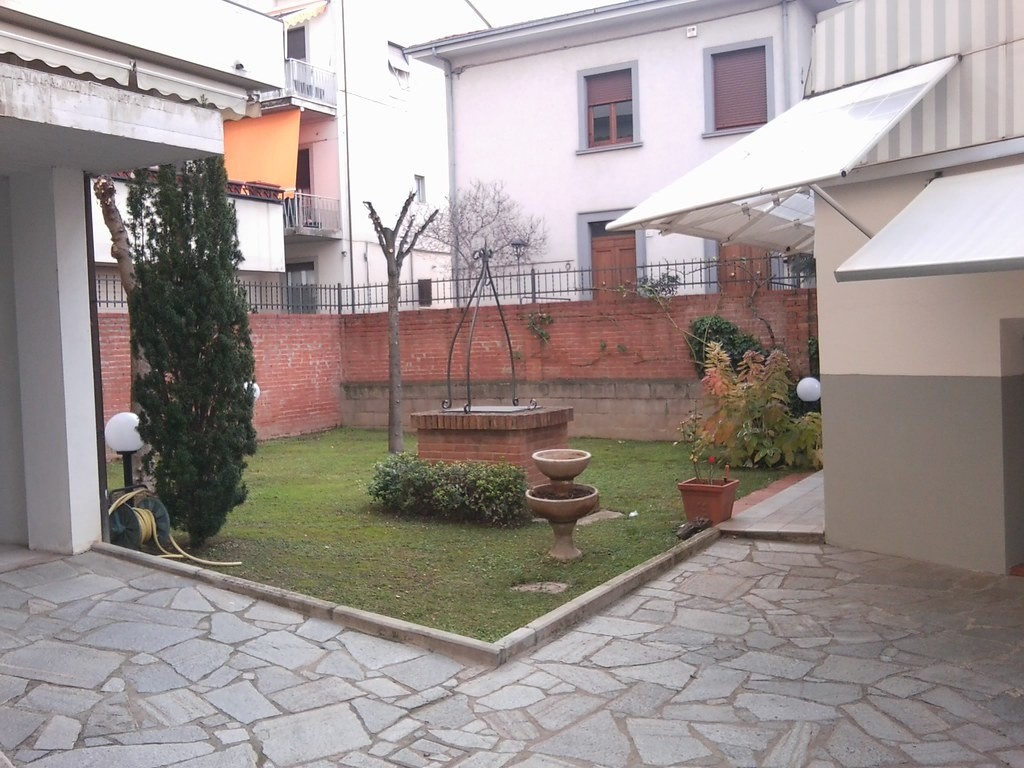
[834,164,1024,283]
[604,54,963,255]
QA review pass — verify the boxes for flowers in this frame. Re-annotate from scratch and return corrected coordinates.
[674,403,717,485]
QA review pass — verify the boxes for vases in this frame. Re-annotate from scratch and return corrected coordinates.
[679,477,739,527]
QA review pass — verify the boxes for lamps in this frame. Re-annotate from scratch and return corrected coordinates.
[105,412,146,455]
[245,381,261,402]
[797,377,821,402]
